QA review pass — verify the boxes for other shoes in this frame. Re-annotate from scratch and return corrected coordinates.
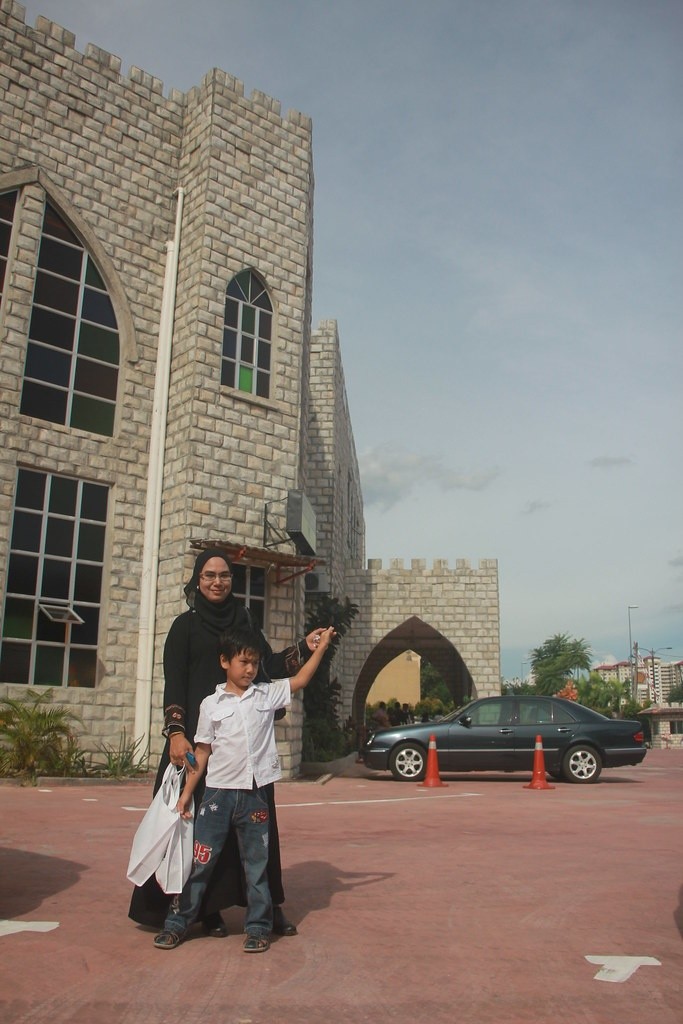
[242,936,268,951]
[154,931,184,948]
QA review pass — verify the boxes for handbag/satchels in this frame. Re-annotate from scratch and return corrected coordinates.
[155,769,196,894]
[127,762,184,886]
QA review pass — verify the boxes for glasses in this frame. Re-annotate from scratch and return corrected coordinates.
[200,572,233,580]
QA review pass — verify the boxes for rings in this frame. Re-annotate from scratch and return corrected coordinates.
[182,760,184,763]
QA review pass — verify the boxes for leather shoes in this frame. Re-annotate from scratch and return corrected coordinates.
[272,907,297,935]
[202,913,226,937]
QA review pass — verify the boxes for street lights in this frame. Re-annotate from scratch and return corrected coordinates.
[628,605,639,700]
[632,646,673,703]
[520,662,527,685]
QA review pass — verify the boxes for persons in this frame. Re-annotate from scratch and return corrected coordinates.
[127,548,339,937]
[151,625,338,951]
[370,699,430,727]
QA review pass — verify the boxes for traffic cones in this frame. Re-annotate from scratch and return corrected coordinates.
[417,734,449,787]
[522,735,557,790]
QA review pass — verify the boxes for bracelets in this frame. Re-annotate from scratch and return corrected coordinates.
[168,732,184,737]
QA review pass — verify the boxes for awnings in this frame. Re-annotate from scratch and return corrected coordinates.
[188,538,328,587]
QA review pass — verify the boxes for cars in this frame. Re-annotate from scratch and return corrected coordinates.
[364,695,647,784]
